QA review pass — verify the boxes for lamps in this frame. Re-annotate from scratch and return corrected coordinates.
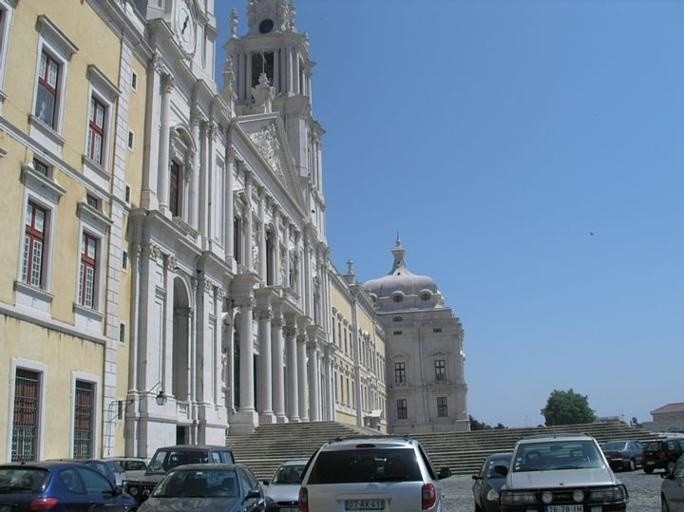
[109,381,168,422]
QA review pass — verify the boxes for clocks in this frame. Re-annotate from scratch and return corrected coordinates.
[174,1,198,55]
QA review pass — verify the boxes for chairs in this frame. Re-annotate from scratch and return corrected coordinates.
[187,478,239,497]
[521,449,589,467]
[353,460,405,478]
[490,465,508,477]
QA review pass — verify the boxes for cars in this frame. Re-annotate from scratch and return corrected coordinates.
[263,460,309,512]
[298,435,451,512]
[0,445,264,512]
[495,433,629,512]
[471,452,513,512]
[602,438,684,512]
